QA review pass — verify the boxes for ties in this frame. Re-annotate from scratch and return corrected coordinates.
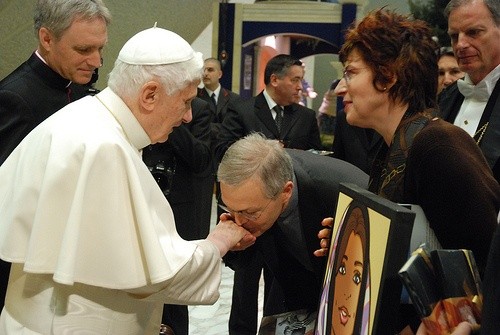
[273,104,284,134]
[210,93,217,111]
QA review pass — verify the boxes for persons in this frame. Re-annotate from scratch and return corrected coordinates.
[228,53,323,157]
[434,1,500,184]
[0,21,254,335]
[219,130,369,335]
[418,209,499,335]
[0,0,113,315]
[187,57,242,132]
[431,44,469,105]
[131,97,217,335]
[335,11,500,335]
[317,79,382,172]
[298,60,318,109]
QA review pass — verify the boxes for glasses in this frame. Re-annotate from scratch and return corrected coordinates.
[343,65,374,85]
[217,192,280,224]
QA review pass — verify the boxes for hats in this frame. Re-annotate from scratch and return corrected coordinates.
[118,22,195,65]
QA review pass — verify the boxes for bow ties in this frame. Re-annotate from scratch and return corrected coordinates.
[457,79,492,101]
